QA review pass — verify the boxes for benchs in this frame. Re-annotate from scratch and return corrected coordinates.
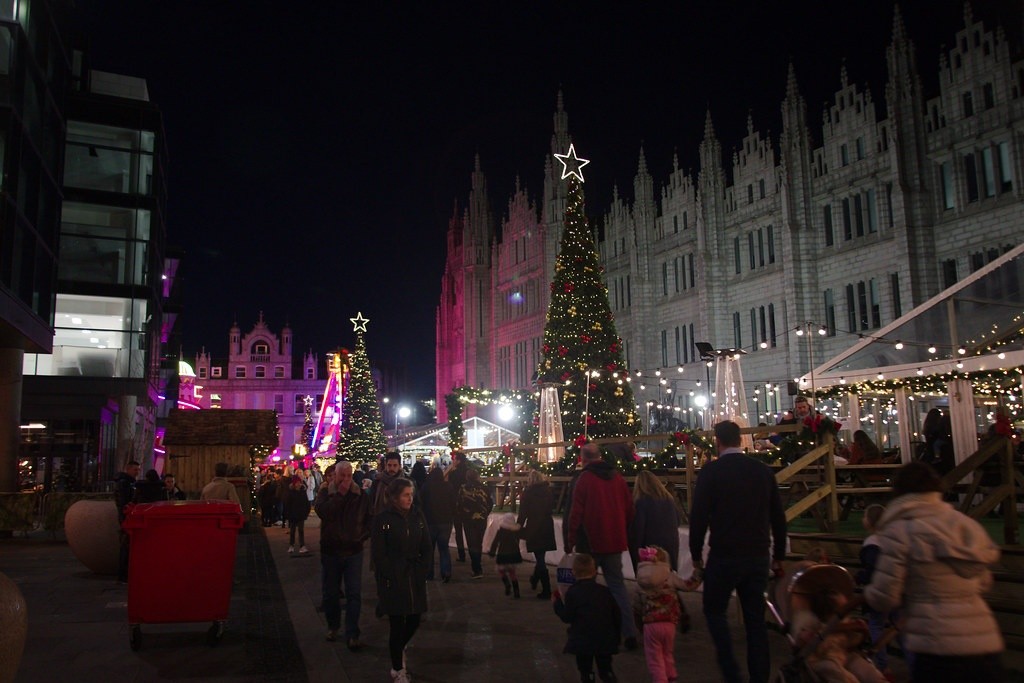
[862,445,901,488]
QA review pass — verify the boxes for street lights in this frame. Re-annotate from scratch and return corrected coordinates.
[394,405,412,450]
[584,368,600,440]
[695,342,715,442]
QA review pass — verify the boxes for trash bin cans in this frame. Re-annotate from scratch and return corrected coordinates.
[121,501,251,651]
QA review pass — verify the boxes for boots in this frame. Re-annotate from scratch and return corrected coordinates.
[503,575,511,596]
[511,579,519,597]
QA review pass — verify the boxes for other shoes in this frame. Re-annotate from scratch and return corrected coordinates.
[288,546,294,552]
[281,523,286,528]
[624,636,638,651]
[681,616,691,634]
[326,623,341,641]
[530,576,537,590]
[348,636,360,650]
[471,571,484,579]
[456,558,465,563]
[299,546,309,553]
[390,648,409,683]
[441,576,450,583]
[537,590,551,600]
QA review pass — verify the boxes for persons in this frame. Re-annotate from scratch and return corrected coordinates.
[791,609,888,683]
[552,554,622,683]
[688,421,786,683]
[628,470,692,634]
[856,504,914,670]
[923,408,1006,519]
[113,461,140,584]
[633,545,704,683]
[147,469,185,500]
[368,453,430,683]
[200,463,243,512]
[258,469,291,533]
[295,465,323,519]
[750,397,882,519]
[489,470,557,598]
[314,460,379,650]
[412,453,493,582]
[865,463,1009,683]
[562,444,638,650]
[287,477,310,553]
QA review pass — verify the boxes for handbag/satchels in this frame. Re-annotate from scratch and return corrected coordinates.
[557,553,575,596]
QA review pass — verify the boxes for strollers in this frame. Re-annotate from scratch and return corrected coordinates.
[762,557,894,683]
